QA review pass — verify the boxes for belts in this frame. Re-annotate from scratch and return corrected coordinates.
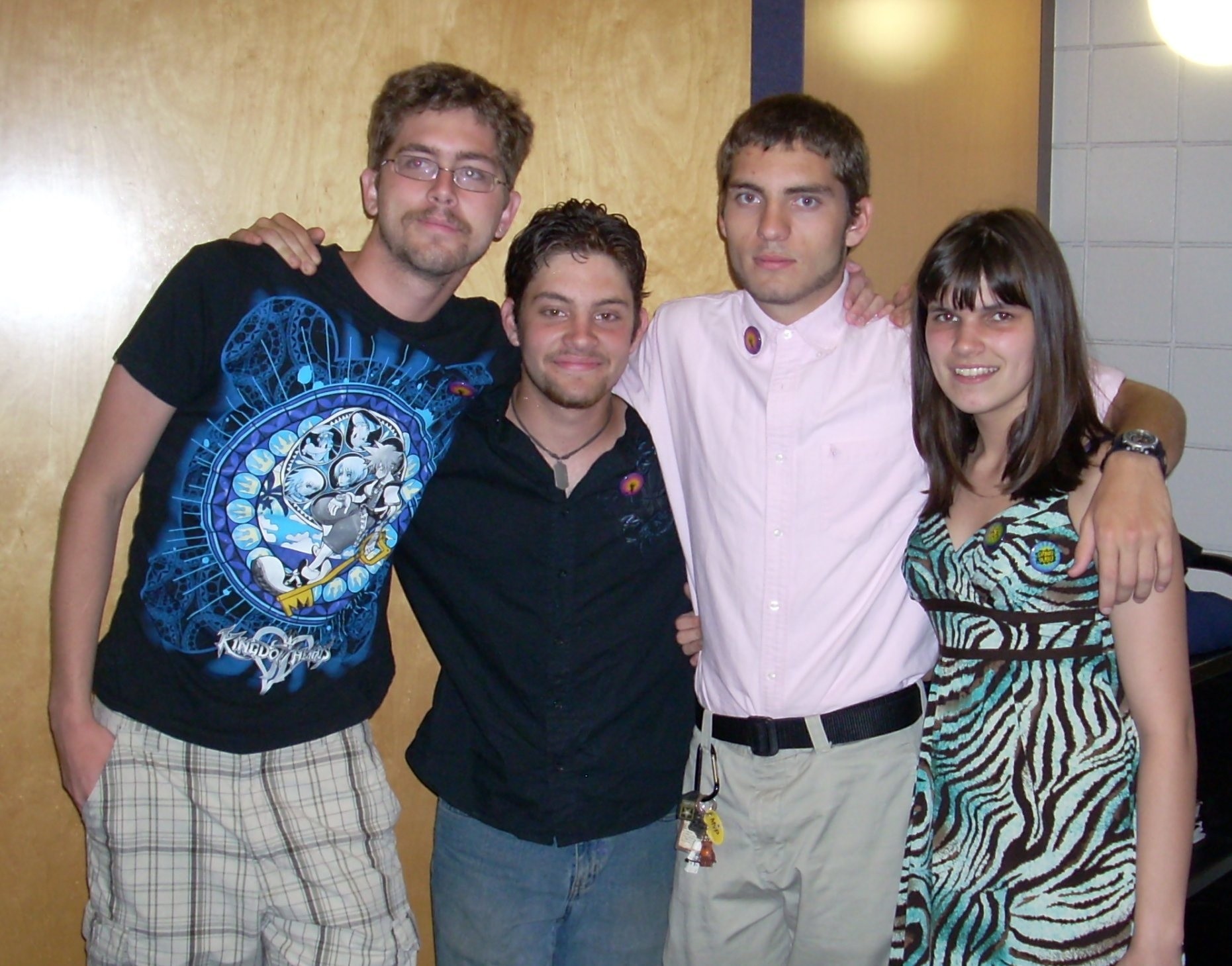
[694,682,923,755]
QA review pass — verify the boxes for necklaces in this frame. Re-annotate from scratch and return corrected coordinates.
[511,394,613,490]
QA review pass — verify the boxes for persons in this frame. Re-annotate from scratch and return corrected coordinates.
[676,208,1199,966]
[232,202,694,966]
[612,94,1186,966]
[46,63,701,966]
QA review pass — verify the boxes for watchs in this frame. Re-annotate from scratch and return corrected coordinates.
[1100,429,1168,478]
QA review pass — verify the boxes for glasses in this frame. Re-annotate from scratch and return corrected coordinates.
[373,154,510,194]
